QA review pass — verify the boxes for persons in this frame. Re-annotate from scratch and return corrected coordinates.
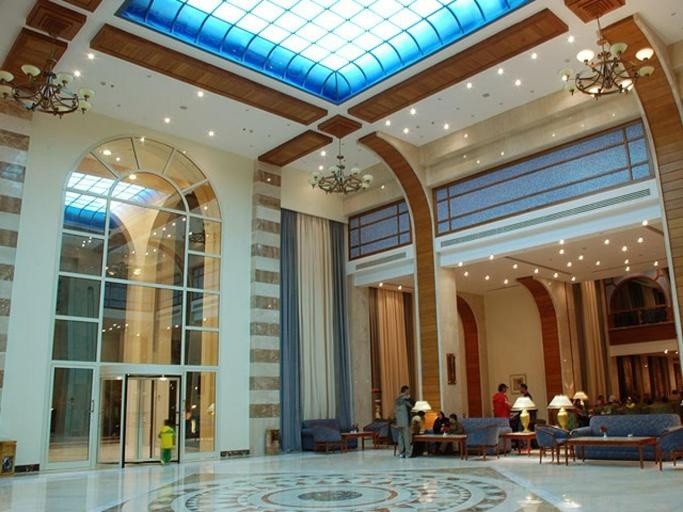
[158,419,176,465]
[391,383,683,459]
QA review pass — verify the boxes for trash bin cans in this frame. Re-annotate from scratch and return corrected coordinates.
[0,441,16,476]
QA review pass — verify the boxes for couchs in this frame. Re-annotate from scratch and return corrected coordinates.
[424,418,512,455]
[569,414,681,461]
[302,418,359,450]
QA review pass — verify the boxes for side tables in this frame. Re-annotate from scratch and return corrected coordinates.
[499,431,536,456]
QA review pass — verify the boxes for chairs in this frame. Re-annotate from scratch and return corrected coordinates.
[362,418,397,449]
[390,423,399,455]
[312,425,340,452]
[534,424,575,465]
[465,423,500,460]
[656,426,683,471]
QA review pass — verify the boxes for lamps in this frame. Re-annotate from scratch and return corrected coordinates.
[510,396,538,432]
[306,135,373,194]
[573,391,588,410]
[545,395,575,432]
[411,401,432,416]
[0,21,94,118]
[561,15,655,99]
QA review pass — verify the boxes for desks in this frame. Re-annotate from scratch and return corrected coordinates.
[340,432,380,450]
[410,433,466,460]
[566,436,657,469]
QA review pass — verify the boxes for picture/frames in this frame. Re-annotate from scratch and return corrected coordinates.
[510,374,527,394]
